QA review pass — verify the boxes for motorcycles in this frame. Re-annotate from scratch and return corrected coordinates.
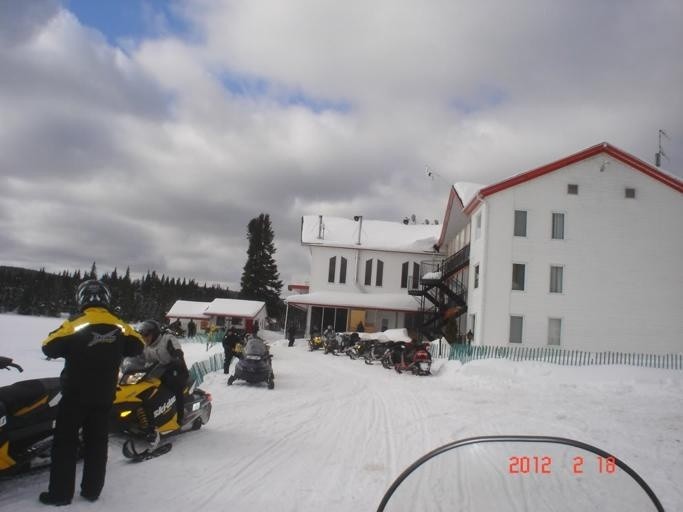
[308,322,431,376]
[0,356,87,479]
[164,325,271,357]
[227,338,273,390]
[110,353,211,461]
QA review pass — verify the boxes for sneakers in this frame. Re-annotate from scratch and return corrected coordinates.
[39,492,71,506]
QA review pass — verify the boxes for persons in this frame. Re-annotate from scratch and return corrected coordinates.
[187,318,194,337]
[38,279,147,506]
[309,325,320,340]
[357,321,364,331]
[174,318,181,326]
[323,324,337,344]
[135,320,192,452]
[222,329,243,374]
[287,324,296,346]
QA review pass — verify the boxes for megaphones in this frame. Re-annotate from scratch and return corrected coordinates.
[354,215,361,221]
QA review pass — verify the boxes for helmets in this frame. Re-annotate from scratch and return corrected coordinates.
[75,280,111,313]
[139,319,161,346]
[122,356,144,373]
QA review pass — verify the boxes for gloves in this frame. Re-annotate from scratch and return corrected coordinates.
[145,430,157,443]
